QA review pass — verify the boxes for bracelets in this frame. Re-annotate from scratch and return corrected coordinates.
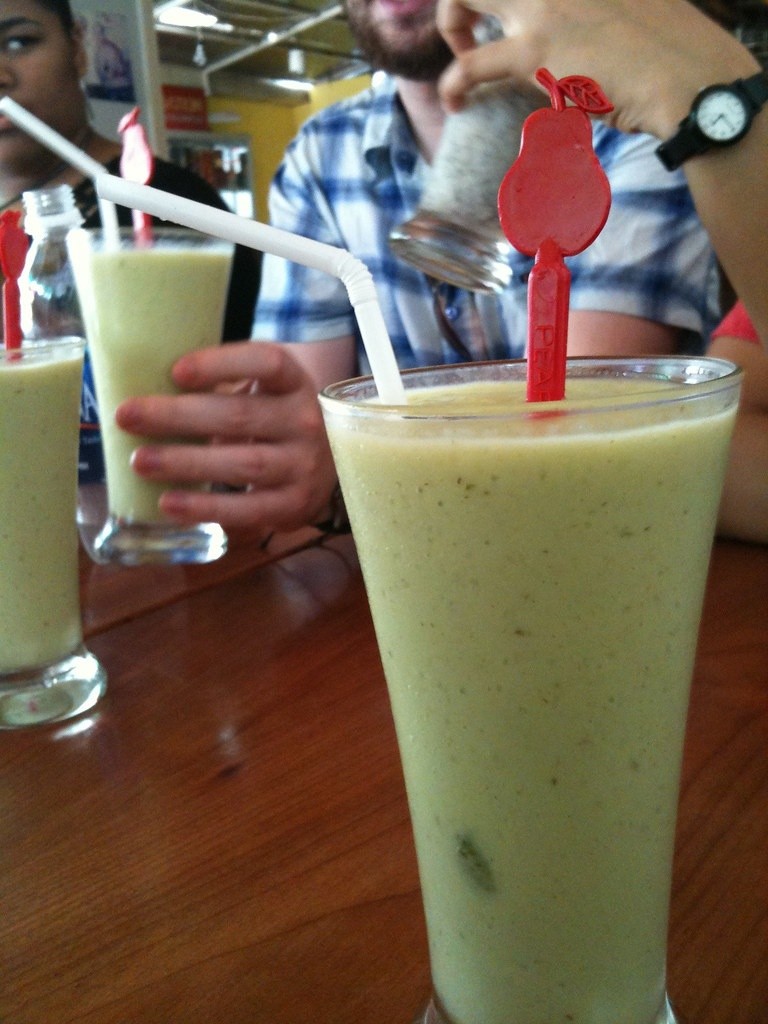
[310,480,351,535]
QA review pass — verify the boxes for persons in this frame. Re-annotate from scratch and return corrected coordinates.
[1,0,768,545]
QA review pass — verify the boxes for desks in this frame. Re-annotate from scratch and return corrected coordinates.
[0,422,768,1024]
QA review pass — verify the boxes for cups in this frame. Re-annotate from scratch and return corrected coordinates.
[59,222,238,568]
[1,338,112,729]
[316,351,744,1024]
[385,17,552,294]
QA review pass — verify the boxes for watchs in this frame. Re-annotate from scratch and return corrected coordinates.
[654,69,768,173]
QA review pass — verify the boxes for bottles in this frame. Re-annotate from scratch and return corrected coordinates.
[15,182,154,567]
